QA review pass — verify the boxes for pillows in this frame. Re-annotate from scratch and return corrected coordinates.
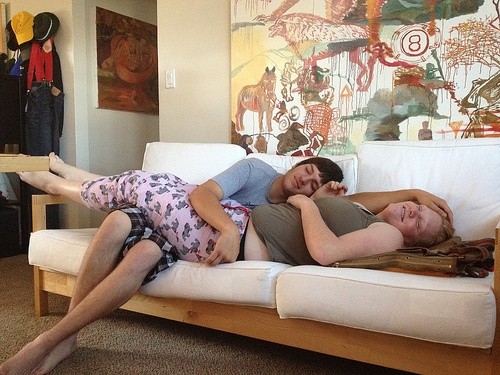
[139,141,246,200]
[247,153,357,194]
[358,138,500,239]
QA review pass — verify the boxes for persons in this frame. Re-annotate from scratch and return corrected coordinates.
[15,151,455,266]
[1,158,456,375]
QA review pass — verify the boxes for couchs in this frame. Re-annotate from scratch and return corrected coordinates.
[28,192,500,375]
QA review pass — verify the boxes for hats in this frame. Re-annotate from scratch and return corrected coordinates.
[6,20,33,51]
[34,12,59,42]
[11,11,34,45]
